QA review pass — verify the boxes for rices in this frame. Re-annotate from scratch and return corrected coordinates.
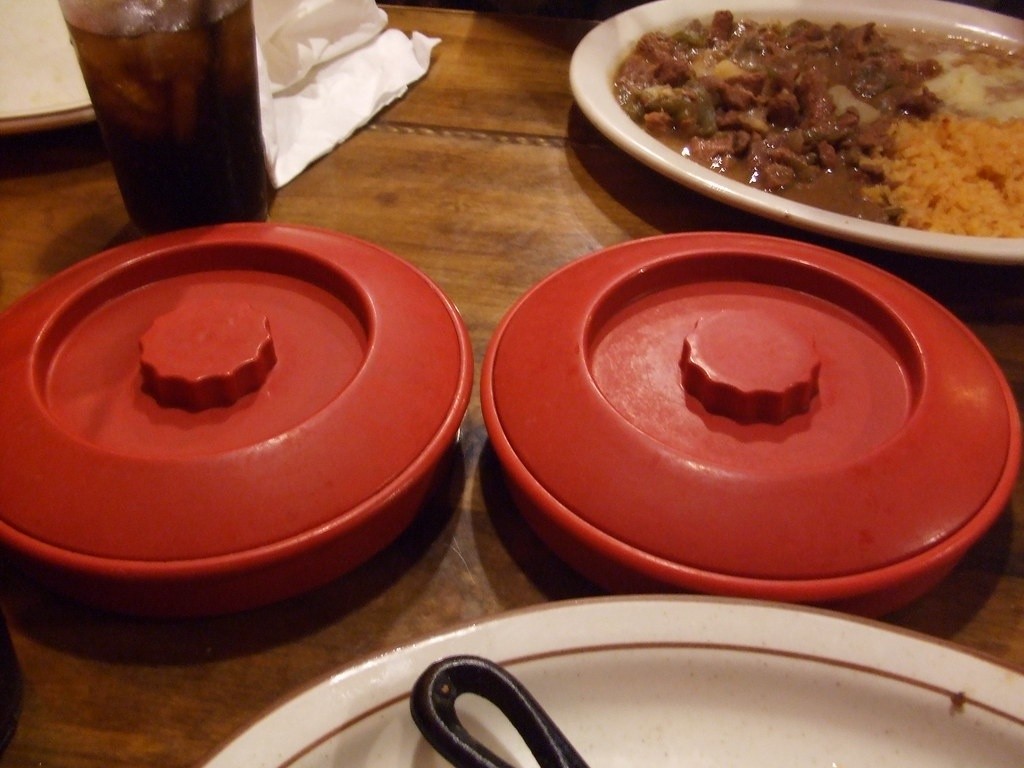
[857,111,1024,239]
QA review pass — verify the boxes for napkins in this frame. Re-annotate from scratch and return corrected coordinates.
[253,0,441,189]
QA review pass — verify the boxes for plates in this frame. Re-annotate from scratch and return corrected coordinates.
[566,0,1024,266]
[193,594,1024,768]
[0,0,96,134]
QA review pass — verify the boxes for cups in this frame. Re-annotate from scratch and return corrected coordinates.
[57,0,276,231]
